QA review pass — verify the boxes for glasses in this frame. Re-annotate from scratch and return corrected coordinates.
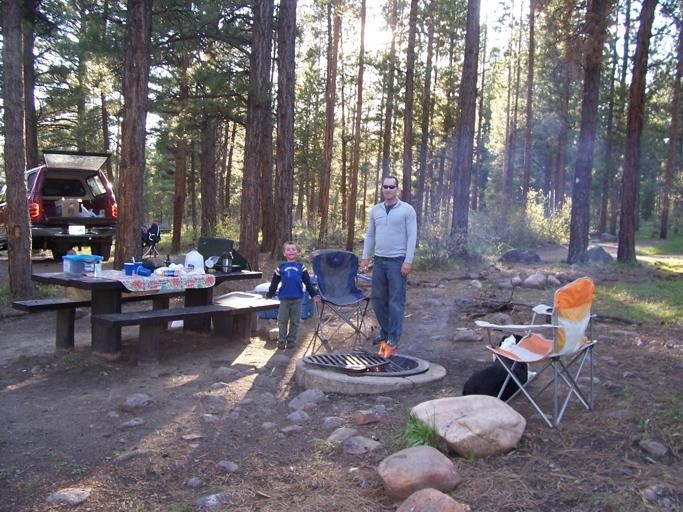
[382,184,396,189]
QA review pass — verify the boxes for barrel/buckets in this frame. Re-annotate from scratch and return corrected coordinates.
[184,246,204,273]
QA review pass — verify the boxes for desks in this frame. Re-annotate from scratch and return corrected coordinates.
[30,269,262,360]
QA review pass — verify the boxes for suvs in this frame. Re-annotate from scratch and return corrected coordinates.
[0,149,120,262]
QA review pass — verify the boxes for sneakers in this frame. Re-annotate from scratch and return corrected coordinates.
[277,341,285,349]
[373,335,385,344]
[287,340,295,348]
[387,339,398,347]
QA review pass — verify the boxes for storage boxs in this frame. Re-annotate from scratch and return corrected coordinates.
[61,254,103,279]
[254,282,314,320]
[54,200,79,217]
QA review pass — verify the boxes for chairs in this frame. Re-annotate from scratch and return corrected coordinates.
[141,222,161,258]
[311,249,371,344]
[474,276,595,429]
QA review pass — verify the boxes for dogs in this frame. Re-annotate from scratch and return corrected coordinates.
[463,333,537,402]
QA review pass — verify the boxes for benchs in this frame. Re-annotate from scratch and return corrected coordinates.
[10,287,184,351]
[89,298,279,367]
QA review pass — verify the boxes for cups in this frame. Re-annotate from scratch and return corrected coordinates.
[124,262,152,277]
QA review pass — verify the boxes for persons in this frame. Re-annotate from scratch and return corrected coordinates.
[265,241,321,349]
[361,175,418,352]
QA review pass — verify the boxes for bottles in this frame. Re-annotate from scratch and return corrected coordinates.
[162,270,179,277]
[222,251,232,274]
